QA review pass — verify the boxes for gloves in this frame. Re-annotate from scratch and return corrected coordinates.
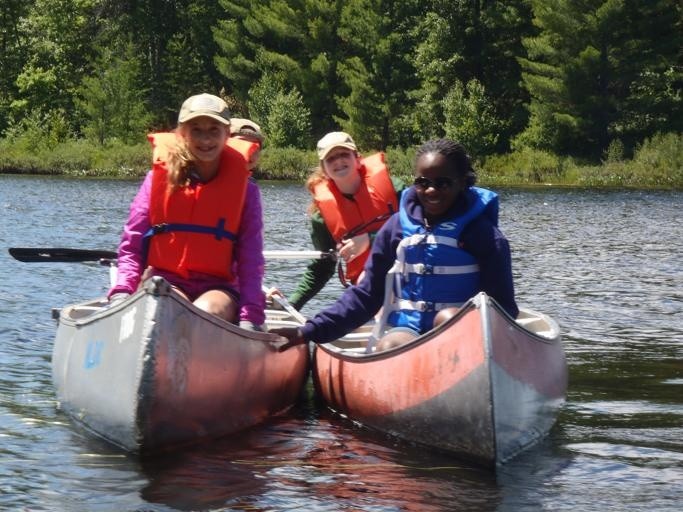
[48,275,312,460]
[307,288,570,473]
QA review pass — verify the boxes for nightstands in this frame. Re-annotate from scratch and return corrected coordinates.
[8,248,336,262]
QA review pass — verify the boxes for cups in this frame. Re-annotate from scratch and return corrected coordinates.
[410,173,461,189]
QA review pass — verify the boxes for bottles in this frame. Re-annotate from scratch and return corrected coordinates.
[176,93,229,126]
[228,118,263,138]
[316,130,356,160]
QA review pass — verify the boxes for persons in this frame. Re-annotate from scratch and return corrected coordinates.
[266,129,397,313]
[268,140,519,353]
[112,94,266,332]
